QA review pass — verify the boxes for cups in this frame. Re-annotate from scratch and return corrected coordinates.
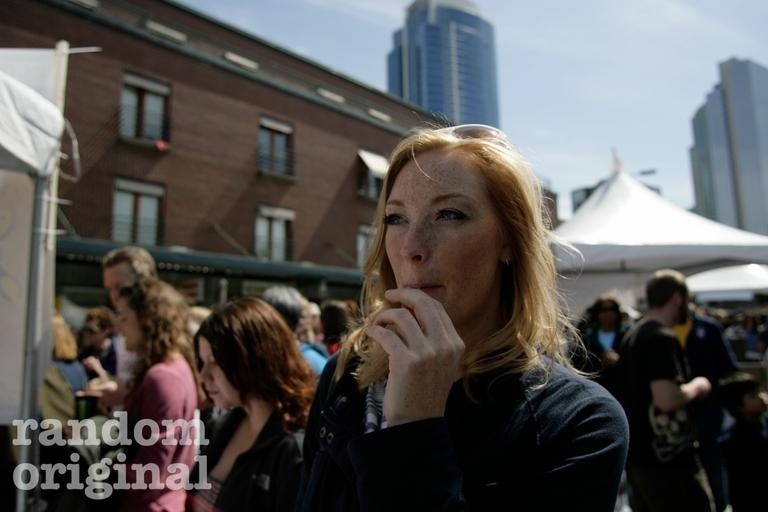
[73,391,100,420]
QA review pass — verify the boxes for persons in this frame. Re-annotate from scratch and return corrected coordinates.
[37,244,766,511]
[296,123,633,510]
